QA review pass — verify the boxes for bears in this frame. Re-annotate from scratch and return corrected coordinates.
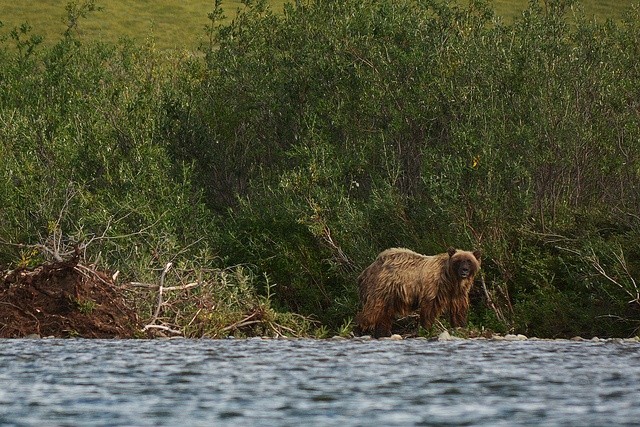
[355,246,484,339]
